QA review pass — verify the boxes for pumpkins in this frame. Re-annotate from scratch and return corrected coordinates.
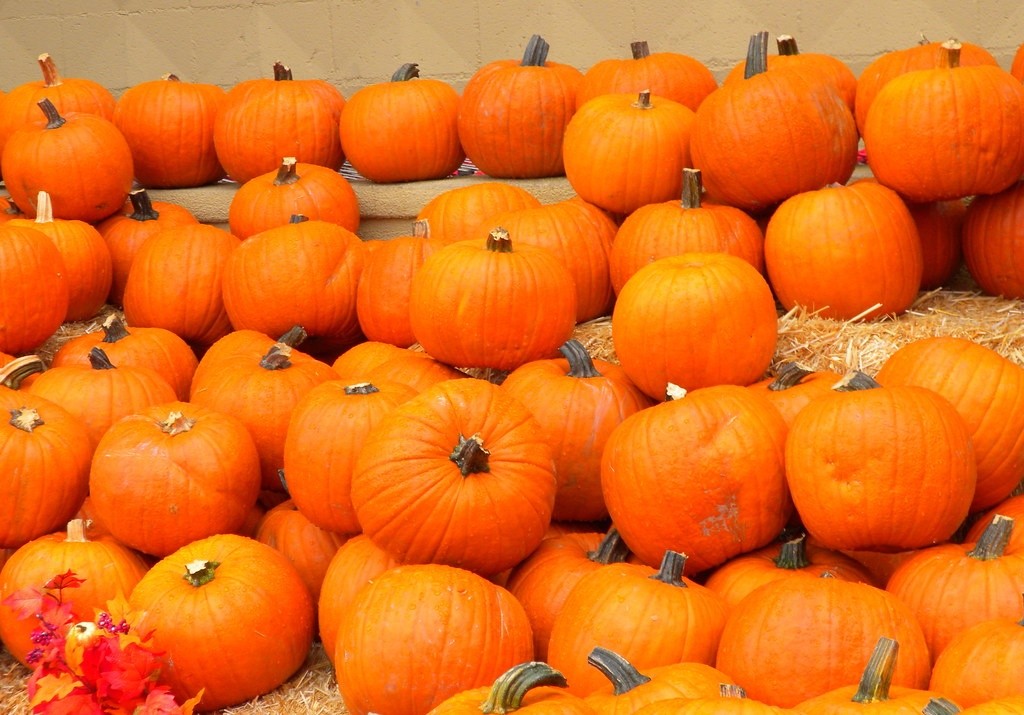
[0,31,1024,715]
